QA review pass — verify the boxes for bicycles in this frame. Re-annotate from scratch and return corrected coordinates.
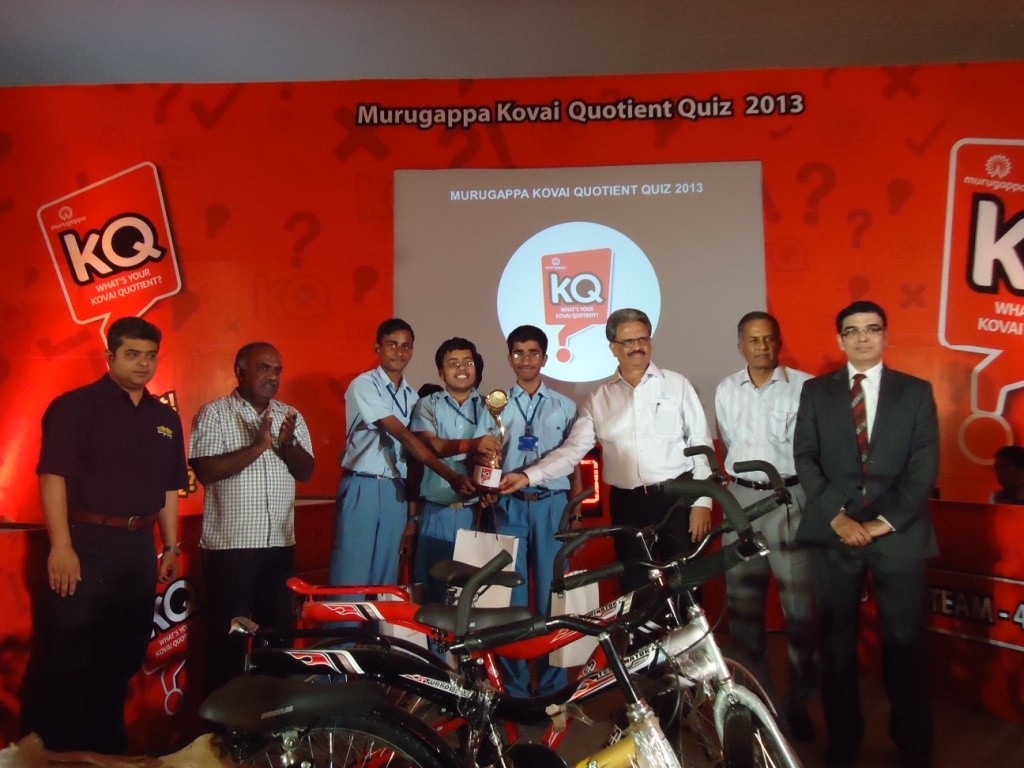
[199,444,806,768]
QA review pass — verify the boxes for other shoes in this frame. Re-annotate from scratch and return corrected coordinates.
[786,706,814,742]
[825,735,857,768]
[903,744,933,768]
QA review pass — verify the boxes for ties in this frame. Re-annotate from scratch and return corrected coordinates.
[850,372,870,506]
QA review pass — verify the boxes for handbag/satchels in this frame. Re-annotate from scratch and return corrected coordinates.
[448,505,519,609]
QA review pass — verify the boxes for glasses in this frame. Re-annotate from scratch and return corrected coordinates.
[513,353,543,361]
[838,327,883,339]
[615,337,653,349]
[441,361,475,369]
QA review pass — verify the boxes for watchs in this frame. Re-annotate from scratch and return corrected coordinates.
[163,545,181,555]
[281,435,299,449]
[408,512,422,522]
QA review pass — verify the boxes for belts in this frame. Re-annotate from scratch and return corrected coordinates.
[67,511,157,533]
[346,471,392,480]
[512,489,564,501]
[636,479,671,494]
[430,501,480,510]
[730,477,800,491]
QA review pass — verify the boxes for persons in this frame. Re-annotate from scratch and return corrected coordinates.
[190,341,316,735]
[20,317,187,756]
[331,300,1024,768]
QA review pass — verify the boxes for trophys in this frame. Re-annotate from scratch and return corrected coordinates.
[471,389,509,488]
